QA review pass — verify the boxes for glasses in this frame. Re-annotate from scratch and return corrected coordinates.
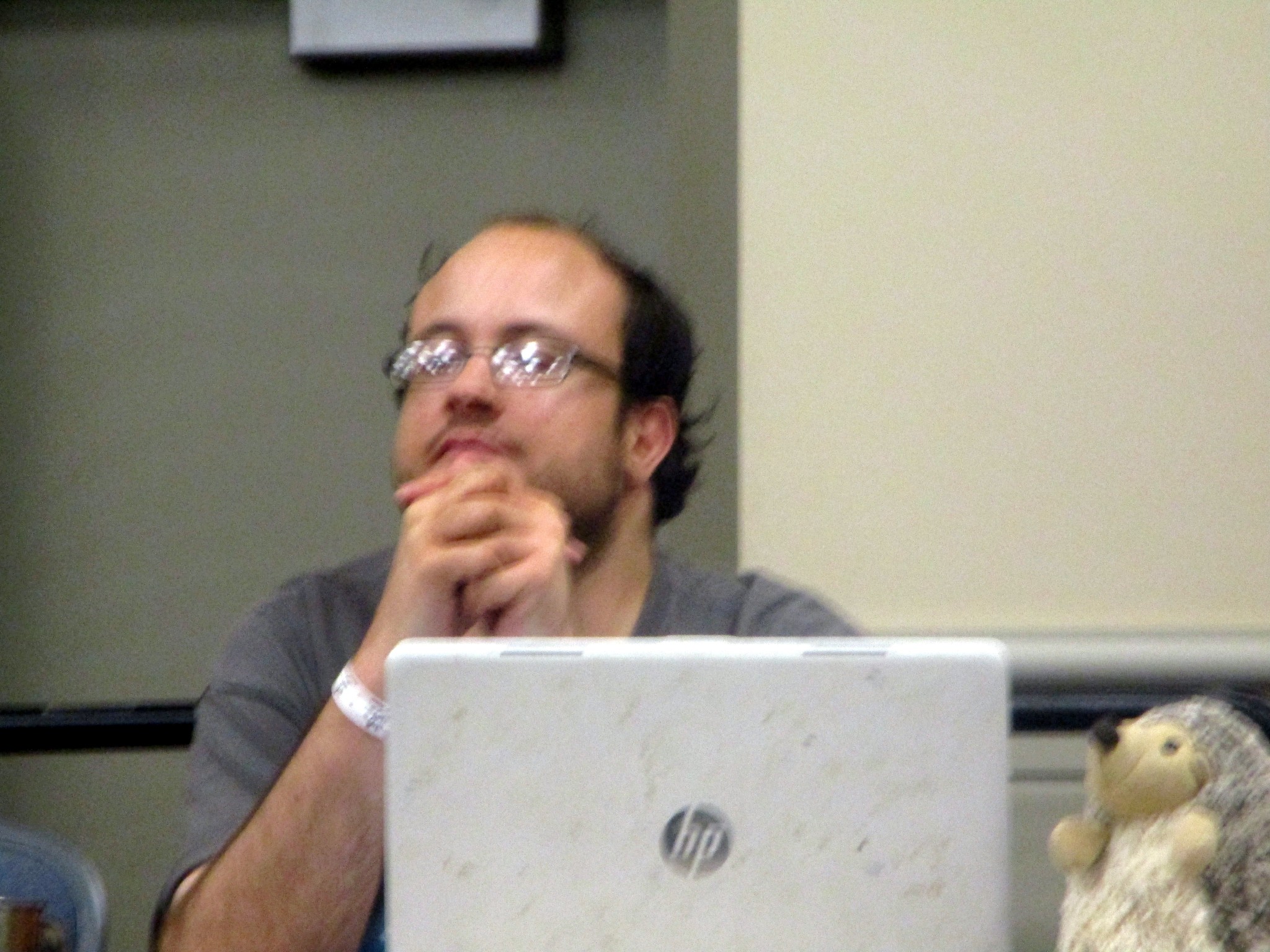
[382,336,628,391]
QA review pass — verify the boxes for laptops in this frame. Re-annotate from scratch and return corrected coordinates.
[384,635,1013,952]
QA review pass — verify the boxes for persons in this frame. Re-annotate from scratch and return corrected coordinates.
[146,213,858,952]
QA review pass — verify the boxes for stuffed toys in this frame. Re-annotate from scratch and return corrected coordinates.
[1043,693,1270,951]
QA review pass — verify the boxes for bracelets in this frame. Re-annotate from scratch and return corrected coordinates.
[330,659,386,743]
